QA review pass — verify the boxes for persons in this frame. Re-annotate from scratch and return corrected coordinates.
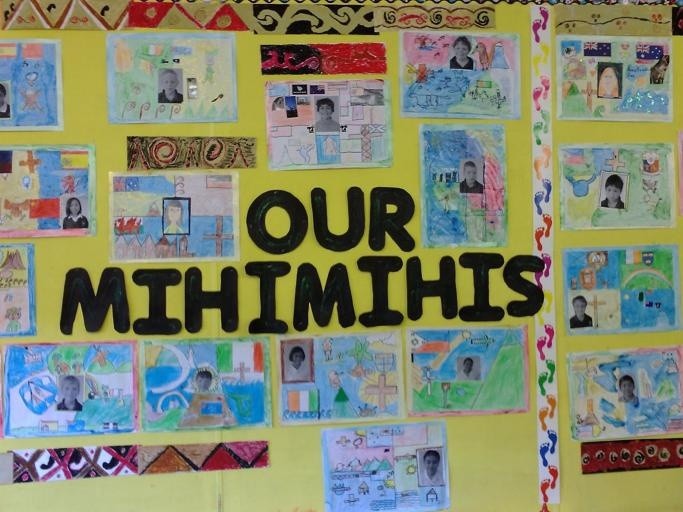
[617,375,639,404]
[601,174,625,209]
[157,68,183,103]
[459,160,483,194]
[57,376,83,411]
[0,83,11,119]
[569,296,593,329]
[457,356,482,381]
[61,197,88,228]
[314,97,340,132]
[419,449,444,487]
[195,370,213,396]
[164,202,186,234]
[448,35,475,71]
[285,344,309,382]
